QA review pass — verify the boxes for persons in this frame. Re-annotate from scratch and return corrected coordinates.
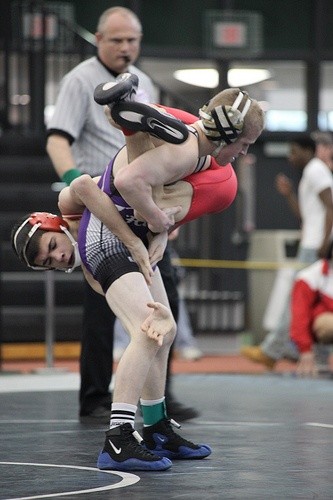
[46,7,200,426]
[12,72,237,346]
[78,88,264,470]
[106,127,332,372]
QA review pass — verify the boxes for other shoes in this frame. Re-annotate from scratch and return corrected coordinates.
[136,398,199,423]
[179,345,203,361]
[79,394,111,430]
[240,340,275,369]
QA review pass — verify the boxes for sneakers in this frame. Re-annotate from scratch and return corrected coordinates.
[96,422,174,473]
[110,101,189,146]
[93,72,140,106]
[140,418,212,460]
